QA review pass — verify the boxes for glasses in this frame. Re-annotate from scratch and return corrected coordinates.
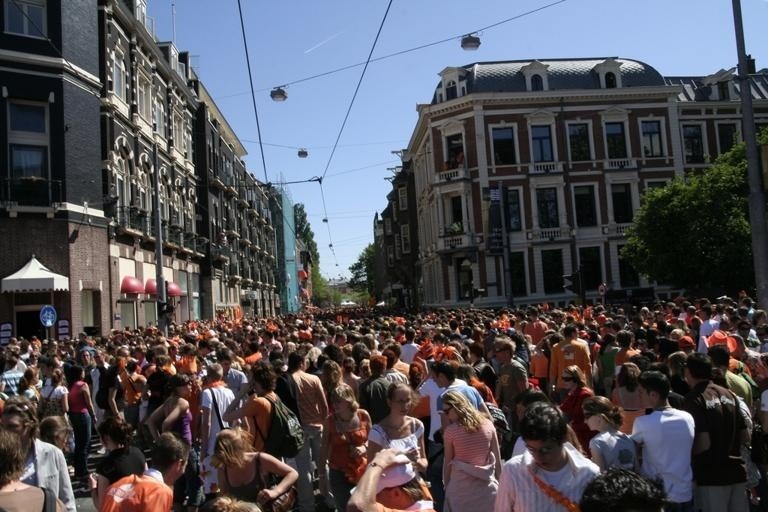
[524,440,560,455]
[389,397,412,405]
[560,374,577,382]
[583,411,600,420]
[443,406,454,414]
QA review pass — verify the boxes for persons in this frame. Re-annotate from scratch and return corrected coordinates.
[0,286,766,511]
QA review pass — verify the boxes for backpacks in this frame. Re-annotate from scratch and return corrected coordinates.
[264,399,305,460]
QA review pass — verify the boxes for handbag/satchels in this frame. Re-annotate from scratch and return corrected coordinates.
[261,481,302,512]
[95,385,110,412]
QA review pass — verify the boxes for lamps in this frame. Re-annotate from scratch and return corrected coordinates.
[296,145,311,161]
[270,85,292,106]
[459,33,483,55]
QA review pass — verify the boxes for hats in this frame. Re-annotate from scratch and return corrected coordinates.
[350,452,417,499]
[701,329,738,354]
[677,335,697,349]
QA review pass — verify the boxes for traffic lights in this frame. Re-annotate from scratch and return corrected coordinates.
[472,286,484,298]
[158,300,168,316]
[562,275,574,294]
